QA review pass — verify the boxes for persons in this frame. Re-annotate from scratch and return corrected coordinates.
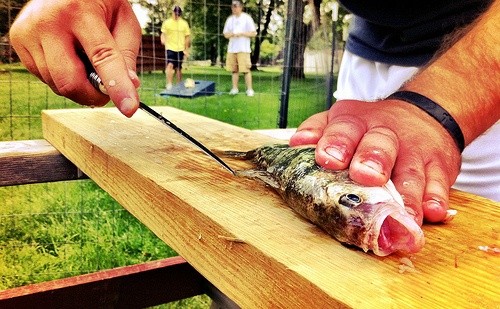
[8,0,500,227]
[223,0,257,96]
[160,6,191,90]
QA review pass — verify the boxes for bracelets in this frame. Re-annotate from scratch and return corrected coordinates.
[383,90,465,154]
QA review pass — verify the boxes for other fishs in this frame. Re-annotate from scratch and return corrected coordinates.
[218,143,425,257]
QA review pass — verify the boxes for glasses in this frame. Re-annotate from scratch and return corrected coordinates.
[174,14,181,16]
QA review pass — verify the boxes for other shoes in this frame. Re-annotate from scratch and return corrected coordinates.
[165,85,172,89]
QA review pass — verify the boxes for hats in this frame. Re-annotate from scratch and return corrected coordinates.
[173,6,182,13]
[231,1,241,7]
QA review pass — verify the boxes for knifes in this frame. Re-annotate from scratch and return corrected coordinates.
[90,72,238,176]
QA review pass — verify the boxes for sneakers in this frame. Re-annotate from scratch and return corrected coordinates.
[246,89,254,96]
[229,89,239,95]
